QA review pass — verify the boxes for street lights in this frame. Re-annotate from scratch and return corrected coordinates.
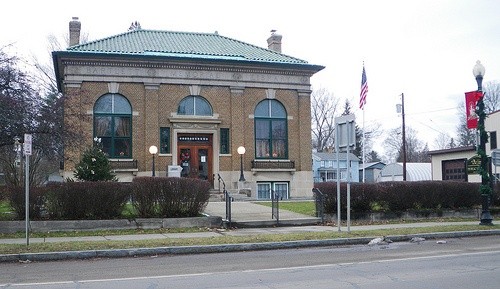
[150,145,158,177]
[237,145,247,182]
[472,60,493,224]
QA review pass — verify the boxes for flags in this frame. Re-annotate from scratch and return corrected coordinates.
[359,65,368,111]
[464,89,479,129]
